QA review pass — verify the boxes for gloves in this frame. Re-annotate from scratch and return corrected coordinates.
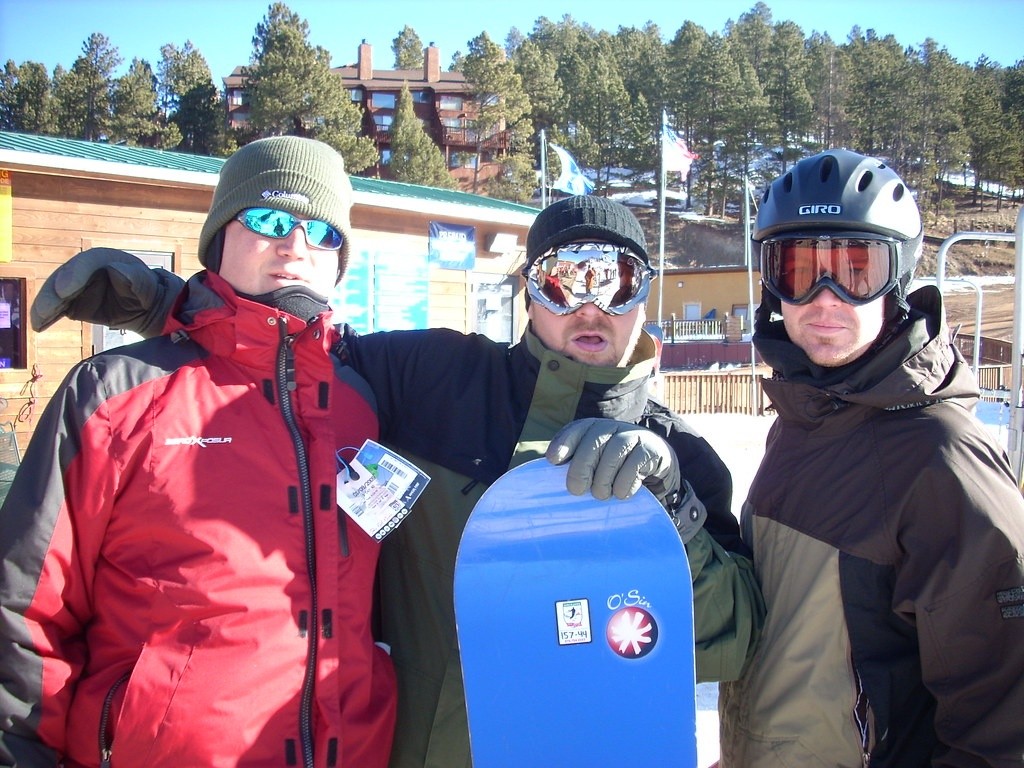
[546,417,708,547]
[31,249,187,340]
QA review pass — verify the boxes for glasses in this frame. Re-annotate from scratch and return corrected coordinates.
[525,241,651,317]
[759,230,897,306]
[229,207,344,250]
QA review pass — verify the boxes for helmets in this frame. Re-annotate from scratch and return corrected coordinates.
[752,148,924,243]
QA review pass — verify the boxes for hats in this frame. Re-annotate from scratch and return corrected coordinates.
[521,195,649,278]
[197,135,350,287]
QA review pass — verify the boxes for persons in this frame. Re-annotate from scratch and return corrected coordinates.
[0,135,398,768]
[718,151,1024,768]
[31,195,764,768]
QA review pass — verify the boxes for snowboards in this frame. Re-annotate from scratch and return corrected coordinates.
[452,455,699,768]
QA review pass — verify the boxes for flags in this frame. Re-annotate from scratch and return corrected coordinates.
[547,142,597,194]
[663,118,697,181]
